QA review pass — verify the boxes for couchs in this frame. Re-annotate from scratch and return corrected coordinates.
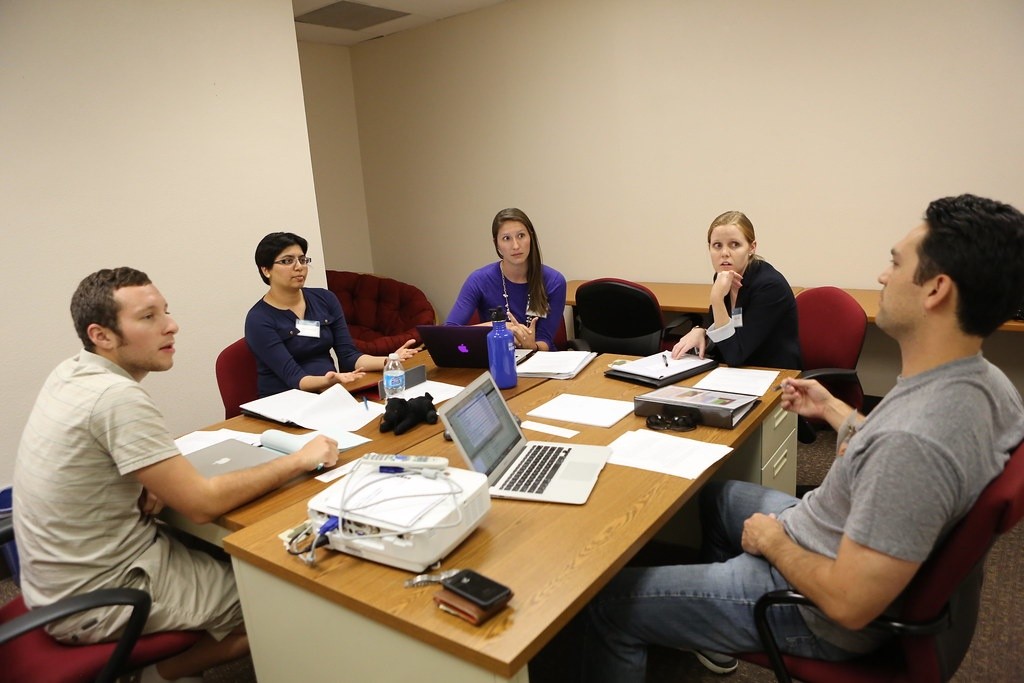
[326,269,434,358]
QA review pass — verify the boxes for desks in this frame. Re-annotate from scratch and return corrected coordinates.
[147,264,1020,682]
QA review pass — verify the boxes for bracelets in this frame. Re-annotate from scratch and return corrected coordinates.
[536,344,538,352]
[384,357,388,367]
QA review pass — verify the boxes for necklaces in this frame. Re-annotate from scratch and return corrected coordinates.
[500,263,530,328]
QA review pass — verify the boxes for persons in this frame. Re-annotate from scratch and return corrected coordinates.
[569,194,1024,683]
[11,267,340,683]
[245,232,418,399]
[671,211,803,372]
[443,208,567,353]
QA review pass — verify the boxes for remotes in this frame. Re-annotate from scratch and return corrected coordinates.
[361,453,449,471]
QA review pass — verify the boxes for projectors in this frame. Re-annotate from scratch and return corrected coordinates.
[306,465,491,573]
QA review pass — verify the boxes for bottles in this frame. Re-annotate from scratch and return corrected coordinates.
[383,353,406,404]
[486,306,518,389]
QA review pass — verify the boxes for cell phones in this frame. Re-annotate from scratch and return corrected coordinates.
[442,569,511,608]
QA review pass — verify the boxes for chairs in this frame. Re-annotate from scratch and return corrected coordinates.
[0,587,203,682]
[215,336,258,420]
[795,284,868,443]
[573,277,703,359]
[468,308,567,350]
[752,438,1023,683]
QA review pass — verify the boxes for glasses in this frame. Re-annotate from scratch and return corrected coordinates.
[273,257,312,265]
[646,414,697,432]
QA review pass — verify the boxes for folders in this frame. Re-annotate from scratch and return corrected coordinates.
[603,350,719,389]
[634,385,763,431]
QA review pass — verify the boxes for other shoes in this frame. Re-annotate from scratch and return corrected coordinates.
[670,646,738,673]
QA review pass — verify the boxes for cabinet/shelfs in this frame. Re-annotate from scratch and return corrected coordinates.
[651,401,799,550]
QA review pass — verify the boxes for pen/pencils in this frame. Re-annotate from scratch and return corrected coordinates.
[362,396,368,411]
[662,354,668,367]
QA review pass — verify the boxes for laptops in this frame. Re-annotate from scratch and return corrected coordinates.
[183,439,283,480]
[436,372,614,506]
[415,324,494,369]
